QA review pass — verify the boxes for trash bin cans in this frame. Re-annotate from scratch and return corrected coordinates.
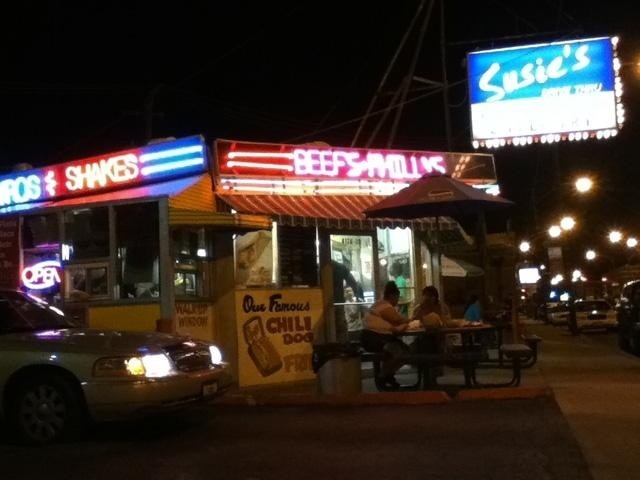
[313,340,362,396]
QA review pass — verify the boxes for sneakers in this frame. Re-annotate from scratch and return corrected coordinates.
[415,376,437,390]
[375,376,400,392]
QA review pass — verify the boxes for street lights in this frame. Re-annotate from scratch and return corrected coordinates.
[609,231,638,271]
[586,250,617,281]
[549,217,577,336]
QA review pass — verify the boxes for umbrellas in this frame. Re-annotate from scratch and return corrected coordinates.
[362,170,516,300]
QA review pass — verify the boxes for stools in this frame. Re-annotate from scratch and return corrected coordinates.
[359,350,386,391]
[452,342,481,383]
[521,333,542,363]
[500,343,531,387]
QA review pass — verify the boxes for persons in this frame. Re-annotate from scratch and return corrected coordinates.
[464,295,489,345]
[359,282,410,391]
[389,262,410,316]
[331,259,366,342]
[407,286,451,388]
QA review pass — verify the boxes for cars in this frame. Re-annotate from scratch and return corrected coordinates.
[0,287,231,446]
[528,297,618,332]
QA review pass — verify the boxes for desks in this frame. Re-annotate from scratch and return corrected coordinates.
[386,319,494,391]
